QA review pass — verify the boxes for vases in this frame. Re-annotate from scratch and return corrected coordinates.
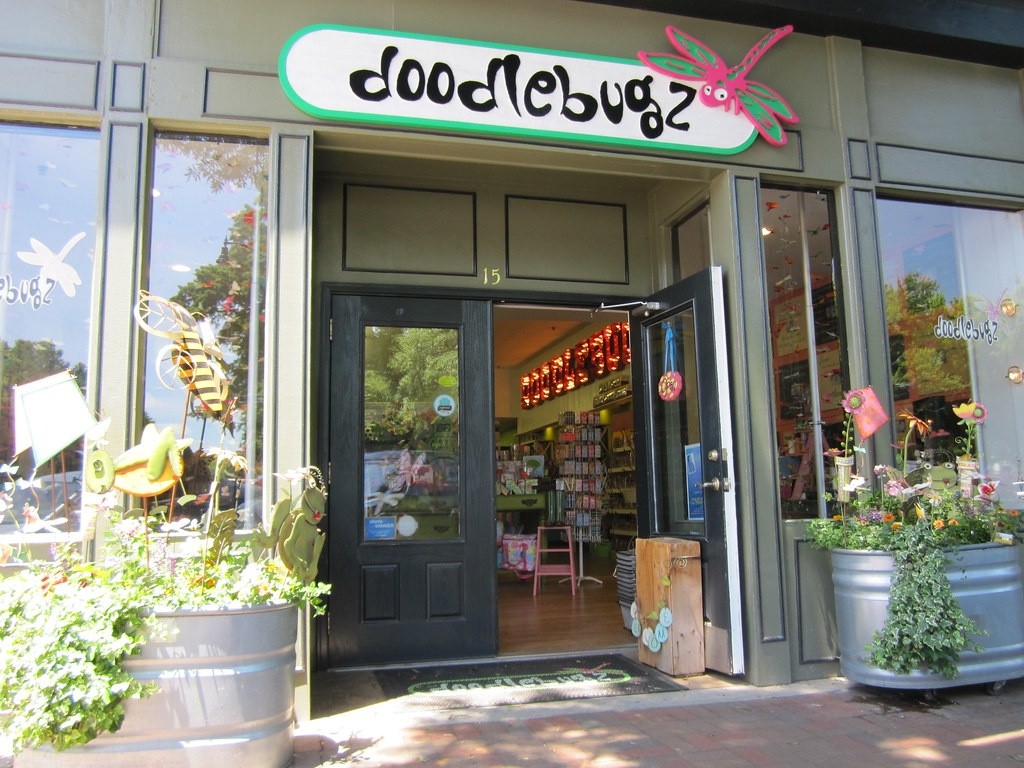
[829,541,1024,702]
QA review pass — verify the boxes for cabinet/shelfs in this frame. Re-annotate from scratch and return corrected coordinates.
[587,395,638,537]
[534,527,577,596]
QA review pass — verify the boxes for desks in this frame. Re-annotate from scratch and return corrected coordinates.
[382,495,548,585]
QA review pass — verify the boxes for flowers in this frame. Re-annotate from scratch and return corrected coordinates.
[802,402,1024,680]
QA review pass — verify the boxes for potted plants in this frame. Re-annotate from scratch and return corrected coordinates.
[0,425,332,768]
